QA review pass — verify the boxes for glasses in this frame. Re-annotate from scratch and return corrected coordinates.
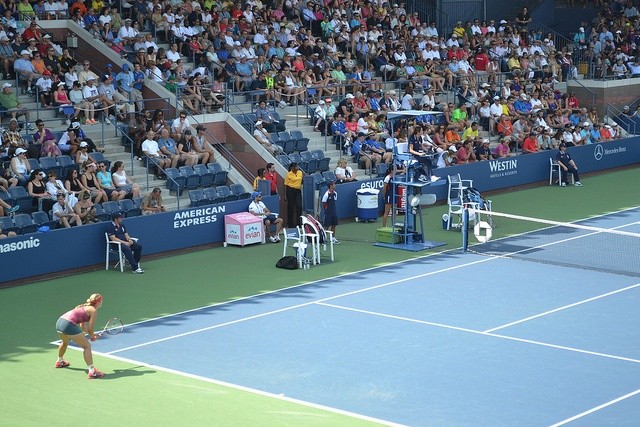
[360,38,365,40]
[78,11,81,12]
[475,21,479,23]
[452,58,456,60]
[77,85,80,87]
[328,51,333,53]
[83,64,89,66]
[159,114,162,116]
[231,19,235,21]
[181,116,185,118]
[74,171,76,174]
[39,173,43,176]
[373,27,376,29]
[259,195,262,198]
[58,193,63,195]
[48,175,53,178]
[493,43,497,45]
[327,101,331,103]
[82,146,88,148]
[99,165,104,168]
[39,123,45,126]
[94,23,97,25]
[83,196,90,200]
[50,49,54,52]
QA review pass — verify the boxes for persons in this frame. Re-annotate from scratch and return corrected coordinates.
[248,191,283,242]
[422,127,449,159]
[321,181,342,244]
[554,142,583,187]
[440,128,512,165]
[314,89,411,174]
[275,0,501,95]
[0,0,227,131]
[619,104,640,119]
[131,109,215,179]
[253,168,268,194]
[573,0,640,80]
[265,162,281,200]
[108,211,145,274]
[459,75,622,154]
[108,0,384,107]
[403,88,468,130]
[500,6,576,81]
[0,227,17,239]
[410,125,436,184]
[255,101,285,132]
[1,117,140,229]
[54,292,106,379]
[139,187,167,215]
[383,165,399,225]
[283,161,305,226]
[254,120,282,155]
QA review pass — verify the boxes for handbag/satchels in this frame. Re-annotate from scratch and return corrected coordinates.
[276,255,298,269]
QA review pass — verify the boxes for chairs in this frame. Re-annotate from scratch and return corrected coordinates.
[376,164,387,178]
[106,233,139,274]
[461,191,493,229]
[550,157,574,187]
[163,162,251,208]
[447,197,465,231]
[283,224,316,269]
[1,128,147,238]
[395,142,430,182]
[378,141,392,153]
[258,213,280,242]
[231,108,339,185]
[119,120,174,154]
[300,214,334,263]
[447,173,473,204]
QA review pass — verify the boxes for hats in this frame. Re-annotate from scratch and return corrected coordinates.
[185,128,192,135]
[43,70,51,75]
[251,191,263,197]
[623,105,630,109]
[106,63,113,67]
[505,78,511,83]
[616,30,621,33]
[583,122,589,126]
[1,36,10,40]
[358,132,366,137]
[31,20,37,25]
[2,82,12,88]
[529,131,538,136]
[122,63,129,70]
[259,25,266,30]
[296,53,301,55]
[226,27,234,31]
[112,210,124,217]
[14,147,28,156]
[336,61,343,66]
[500,19,508,23]
[254,120,262,127]
[493,95,499,100]
[21,49,30,54]
[617,47,621,50]
[43,33,51,38]
[138,48,145,52]
[197,124,207,130]
[28,37,35,41]
[433,43,439,47]
[235,40,241,46]
[565,123,571,128]
[69,121,80,129]
[346,93,355,99]
[314,53,319,56]
[449,146,457,152]
[442,57,446,60]
[482,82,490,87]
[452,33,459,37]
[319,99,325,104]
[559,142,566,146]
[462,80,469,84]
[520,92,526,100]
[579,27,585,32]
[86,74,96,81]
[368,36,375,40]
[604,124,611,128]
[263,40,269,43]
[483,138,489,143]
[326,98,332,102]
[433,57,440,60]
[80,141,88,147]
[57,81,66,86]
[38,171,46,177]
[86,161,96,166]
[73,81,81,86]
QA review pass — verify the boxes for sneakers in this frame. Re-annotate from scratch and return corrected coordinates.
[269,236,276,242]
[418,174,428,182]
[562,182,567,186]
[332,237,341,243]
[274,234,281,242]
[105,118,111,123]
[132,268,144,273]
[56,359,71,367]
[85,119,93,125]
[431,175,441,182]
[88,367,104,378]
[574,181,583,186]
[92,118,97,124]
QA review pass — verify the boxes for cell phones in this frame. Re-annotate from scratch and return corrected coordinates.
[90,204,95,211]
[161,206,163,210]
